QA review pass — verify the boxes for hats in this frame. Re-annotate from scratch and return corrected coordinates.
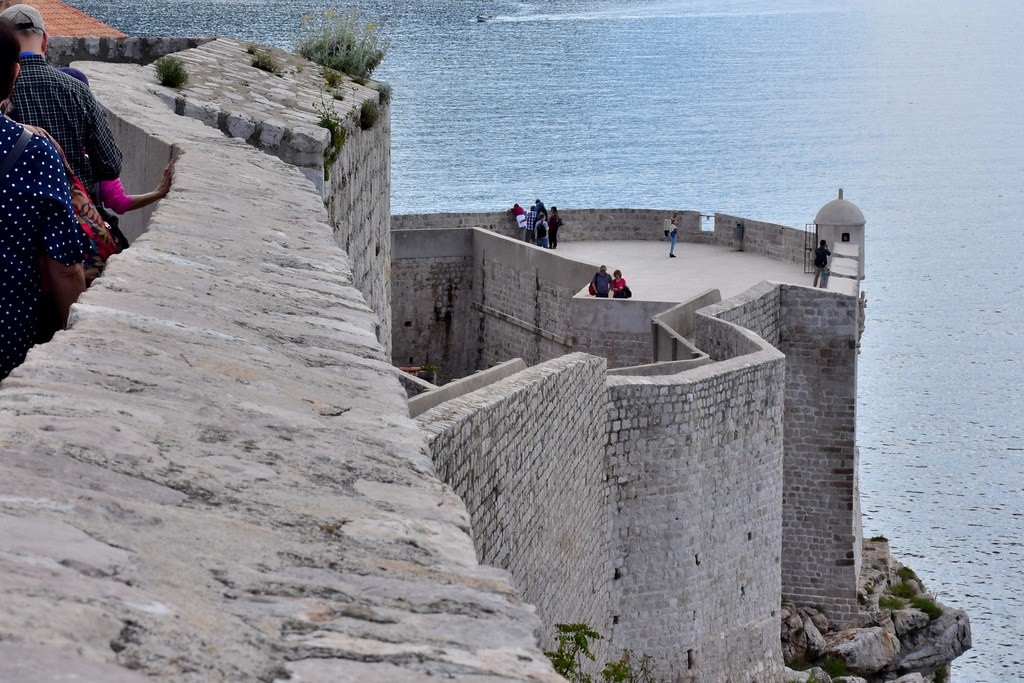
[0,4,44,33]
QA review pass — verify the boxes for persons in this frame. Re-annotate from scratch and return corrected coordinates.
[0,3,175,381]
[611,270,626,298]
[549,207,559,249]
[534,213,549,248]
[669,219,681,258]
[592,265,612,297]
[511,199,547,244]
[813,240,831,288]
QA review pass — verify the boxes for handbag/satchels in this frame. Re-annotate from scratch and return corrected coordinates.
[588,272,598,295]
[624,285,632,299]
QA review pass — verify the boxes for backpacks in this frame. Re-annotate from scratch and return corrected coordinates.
[537,220,546,238]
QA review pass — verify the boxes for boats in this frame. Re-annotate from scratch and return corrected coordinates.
[477,9,492,21]
